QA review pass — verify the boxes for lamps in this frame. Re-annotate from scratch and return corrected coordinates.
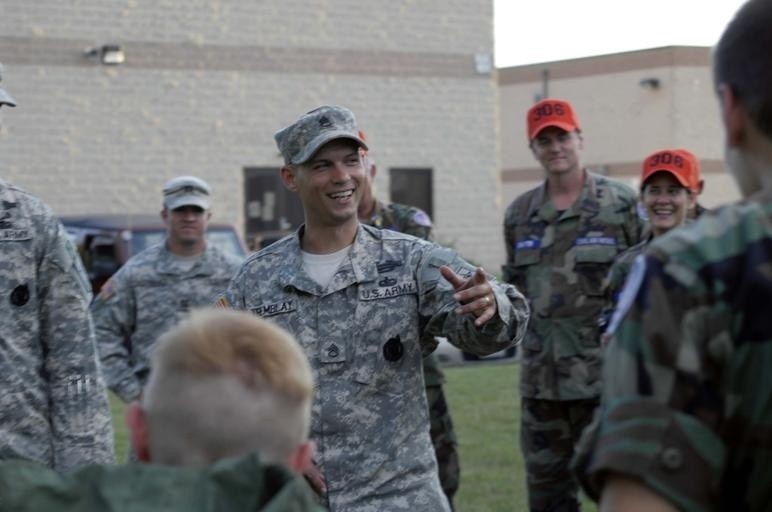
[83,43,126,64]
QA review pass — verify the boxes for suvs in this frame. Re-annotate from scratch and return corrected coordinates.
[61,214,251,287]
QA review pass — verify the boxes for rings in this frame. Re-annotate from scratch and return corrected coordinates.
[484,296,490,305]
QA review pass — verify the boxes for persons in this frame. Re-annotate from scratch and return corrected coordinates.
[500,99,646,511]
[358,129,462,510]
[567,0,770,512]
[678,151,707,218]
[218,105,531,511]
[90,177,243,464]
[595,149,693,346]
[1,305,335,511]
[0,88,114,473]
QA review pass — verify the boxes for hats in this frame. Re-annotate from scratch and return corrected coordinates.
[526,98,583,141]
[0,64,18,109]
[161,176,214,211]
[640,148,700,197]
[358,123,370,158]
[274,104,369,168]
